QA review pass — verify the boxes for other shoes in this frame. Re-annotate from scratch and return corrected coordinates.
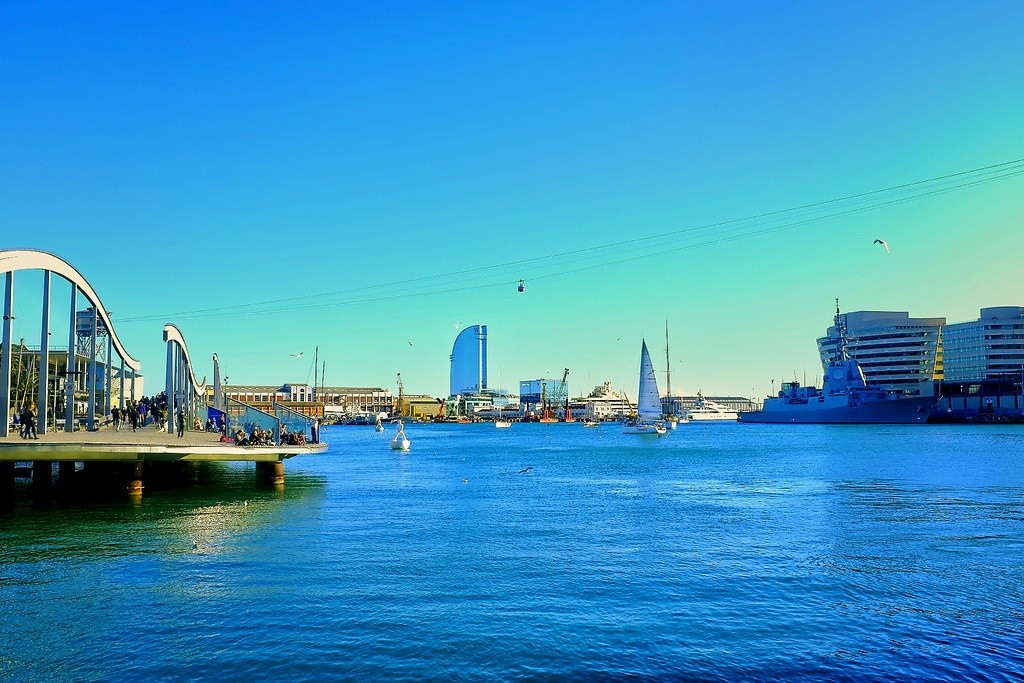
[20,434,27,439]
[29,436,39,440]
[134,430,136,432]
[178,434,183,438]
[163,430,166,432]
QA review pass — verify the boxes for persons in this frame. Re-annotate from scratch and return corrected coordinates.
[106,386,310,449]
[23,403,39,440]
[18,404,33,439]
[47,408,53,426]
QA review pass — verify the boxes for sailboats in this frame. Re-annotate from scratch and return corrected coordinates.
[622,338,664,433]
[496,404,511,427]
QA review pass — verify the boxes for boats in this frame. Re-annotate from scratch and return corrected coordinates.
[337,397,386,425]
[678,412,689,424]
[390,420,410,450]
[375,418,384,431]
[687,390,740,420]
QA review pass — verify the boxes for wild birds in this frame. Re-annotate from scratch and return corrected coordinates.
[463,478,470,482]
[409,340,414,347]
[873,239,890,255]
[519,465,533,474]
[452,321,462,329]
[289,351,304,358]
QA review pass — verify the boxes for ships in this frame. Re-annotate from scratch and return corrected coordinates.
[736,297,944,424]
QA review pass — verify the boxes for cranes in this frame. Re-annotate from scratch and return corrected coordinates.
[396,373,404,417]
[548,368,570,418]
